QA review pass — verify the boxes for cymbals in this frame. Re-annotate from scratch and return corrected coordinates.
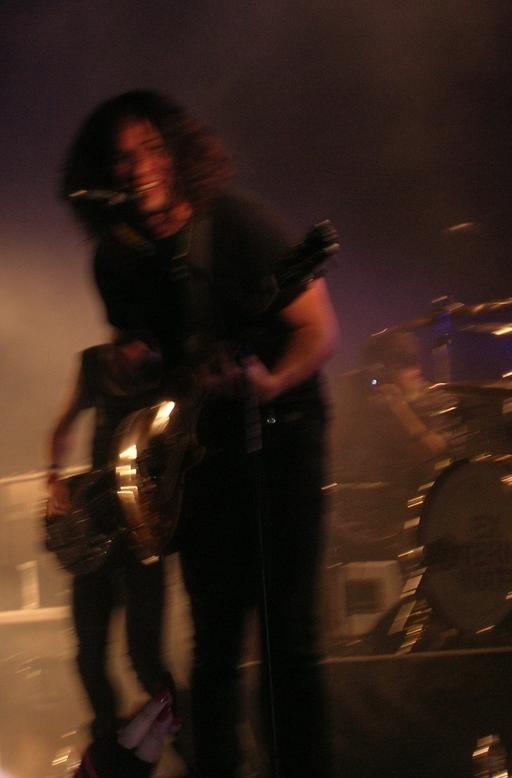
[436,379,512,395]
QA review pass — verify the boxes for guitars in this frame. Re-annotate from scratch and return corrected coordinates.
[113,219,339,558]
[43,470,128,578]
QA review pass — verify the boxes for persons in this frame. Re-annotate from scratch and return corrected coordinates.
[49,332,175,719]
[63,90,339,778]
[340,330,461,577]
[78,692,182,778]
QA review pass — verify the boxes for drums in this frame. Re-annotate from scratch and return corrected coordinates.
[402,457,512,638]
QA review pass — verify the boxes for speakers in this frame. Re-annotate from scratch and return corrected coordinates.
[250,646,511,777]
